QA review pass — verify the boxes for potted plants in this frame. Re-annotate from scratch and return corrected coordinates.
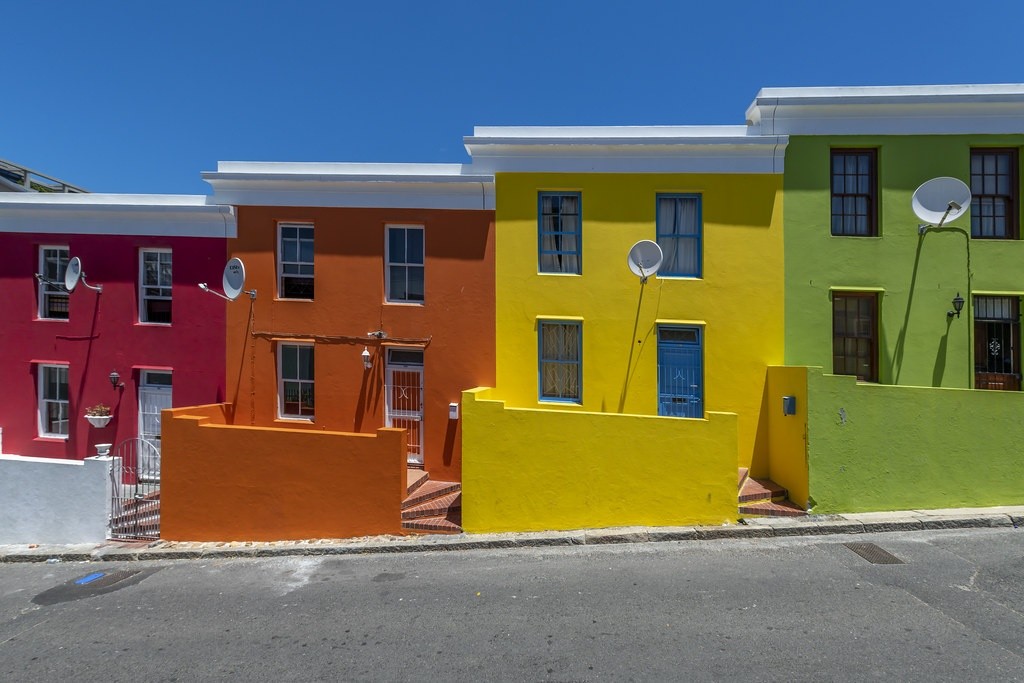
[85,404,113,428]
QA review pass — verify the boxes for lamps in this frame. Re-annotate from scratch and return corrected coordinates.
[362,346,373,370]
[946,292,965,318]
[198,282,234,301]
[34,272,70,294]
[109,369,125,390]
